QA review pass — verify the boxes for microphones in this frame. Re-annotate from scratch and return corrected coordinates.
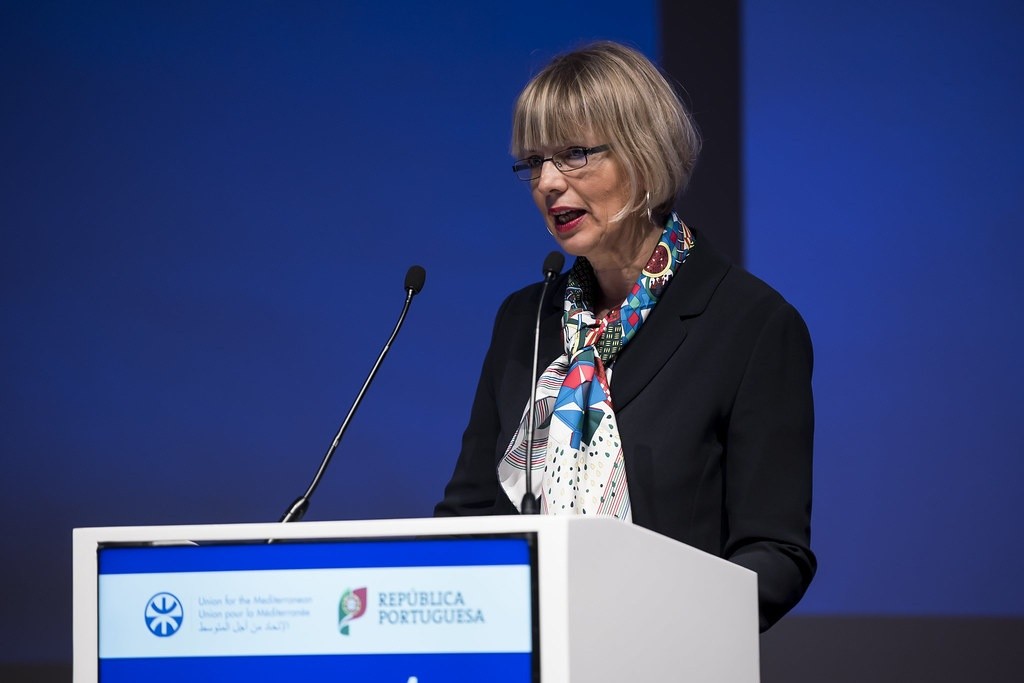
[521,251,566,514]
[280,266,427,524]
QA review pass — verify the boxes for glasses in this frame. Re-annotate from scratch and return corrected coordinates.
[511,143,614,182]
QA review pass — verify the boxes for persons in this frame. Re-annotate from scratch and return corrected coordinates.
[431,42,817,633]
